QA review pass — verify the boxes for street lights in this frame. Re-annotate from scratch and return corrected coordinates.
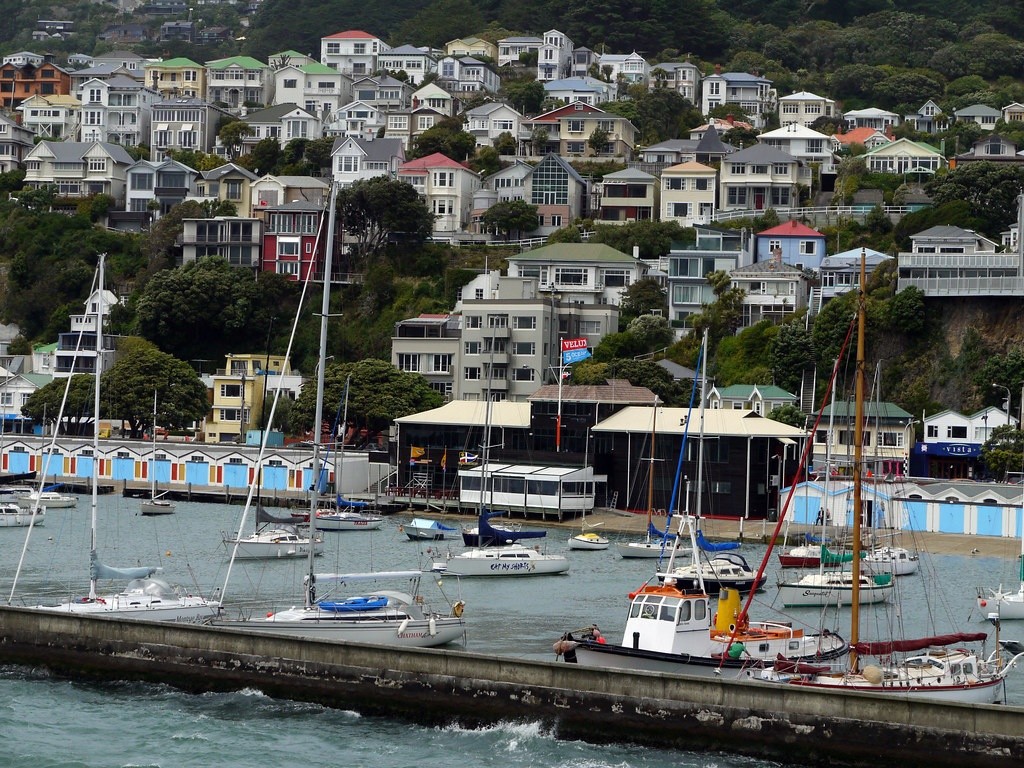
[992,383,1011,443]
[899,419,920,480]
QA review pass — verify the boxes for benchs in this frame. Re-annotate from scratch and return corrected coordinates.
[386,490,449,500]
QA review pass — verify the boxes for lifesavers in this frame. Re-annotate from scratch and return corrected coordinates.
[749,627,762,635]
[737,612,749,632]
[455,602,465,617]
[713,613,717,626]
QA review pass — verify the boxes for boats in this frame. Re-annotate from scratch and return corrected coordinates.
[401,518,459,540]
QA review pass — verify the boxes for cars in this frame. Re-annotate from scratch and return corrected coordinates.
[354,443,386,451]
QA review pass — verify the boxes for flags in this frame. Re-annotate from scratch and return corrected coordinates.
[411,447,425,458]
[459,451,478,464]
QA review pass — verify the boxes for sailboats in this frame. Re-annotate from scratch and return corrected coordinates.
[0,182,480,648]
[561,248,1024,702]
[420,316,570,575]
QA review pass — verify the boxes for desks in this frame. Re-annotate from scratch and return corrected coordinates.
[390,489,446,499]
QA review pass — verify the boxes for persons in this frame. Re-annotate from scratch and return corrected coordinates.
[711,641,751,659]
[816,507,830,525]
